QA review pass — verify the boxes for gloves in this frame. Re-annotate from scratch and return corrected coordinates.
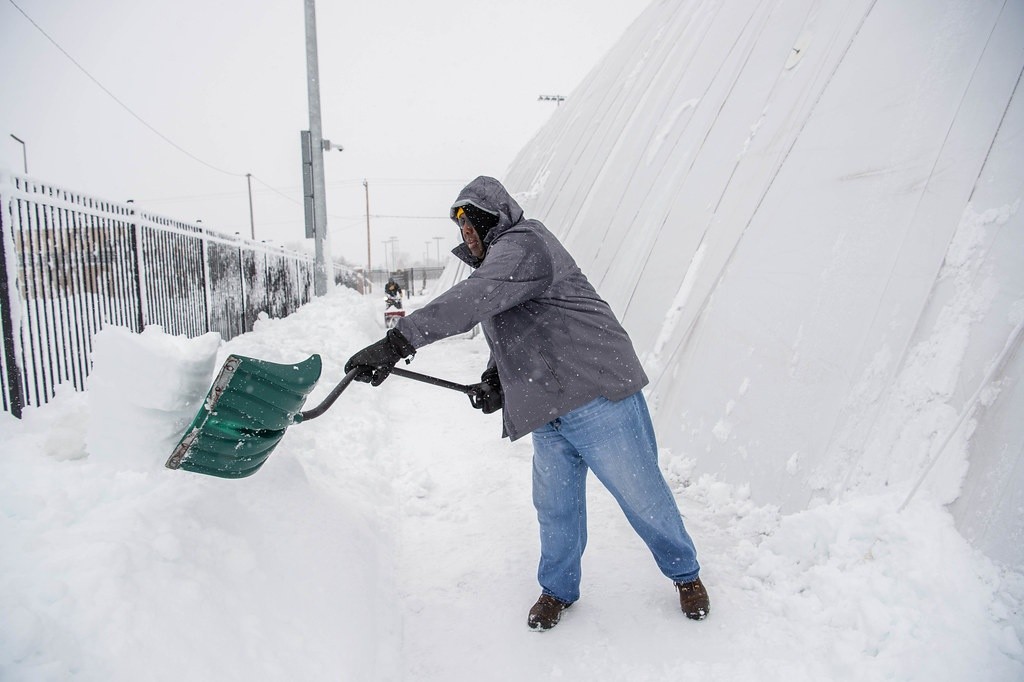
[467,367,504,414]
[344,328,418,387]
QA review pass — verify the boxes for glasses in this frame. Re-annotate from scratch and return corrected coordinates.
[457,216,474,229]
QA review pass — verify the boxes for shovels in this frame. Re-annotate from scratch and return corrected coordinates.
[165,354,495,479]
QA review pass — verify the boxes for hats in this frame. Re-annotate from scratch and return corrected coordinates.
[389,277,393,284]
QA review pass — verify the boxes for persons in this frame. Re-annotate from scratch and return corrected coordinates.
[345,174,712,632]
[385,277,404,301]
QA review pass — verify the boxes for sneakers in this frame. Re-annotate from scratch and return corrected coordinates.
[526,592,573,632]
[677,578,711,621]
